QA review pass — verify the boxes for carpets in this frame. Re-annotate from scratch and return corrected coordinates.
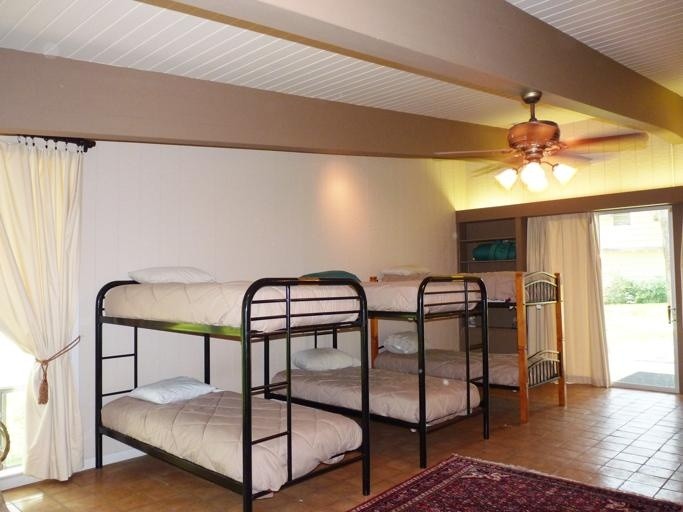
[347,453,683,512]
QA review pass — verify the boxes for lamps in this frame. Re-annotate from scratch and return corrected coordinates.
[494,153,577,193]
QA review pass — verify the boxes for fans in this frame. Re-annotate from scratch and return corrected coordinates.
[433,90,649,177]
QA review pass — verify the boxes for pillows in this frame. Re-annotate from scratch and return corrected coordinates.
[379,264,430,276]
[130,266,214,284]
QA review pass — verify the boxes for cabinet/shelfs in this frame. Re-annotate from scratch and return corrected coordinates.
[457,217,527,354]
